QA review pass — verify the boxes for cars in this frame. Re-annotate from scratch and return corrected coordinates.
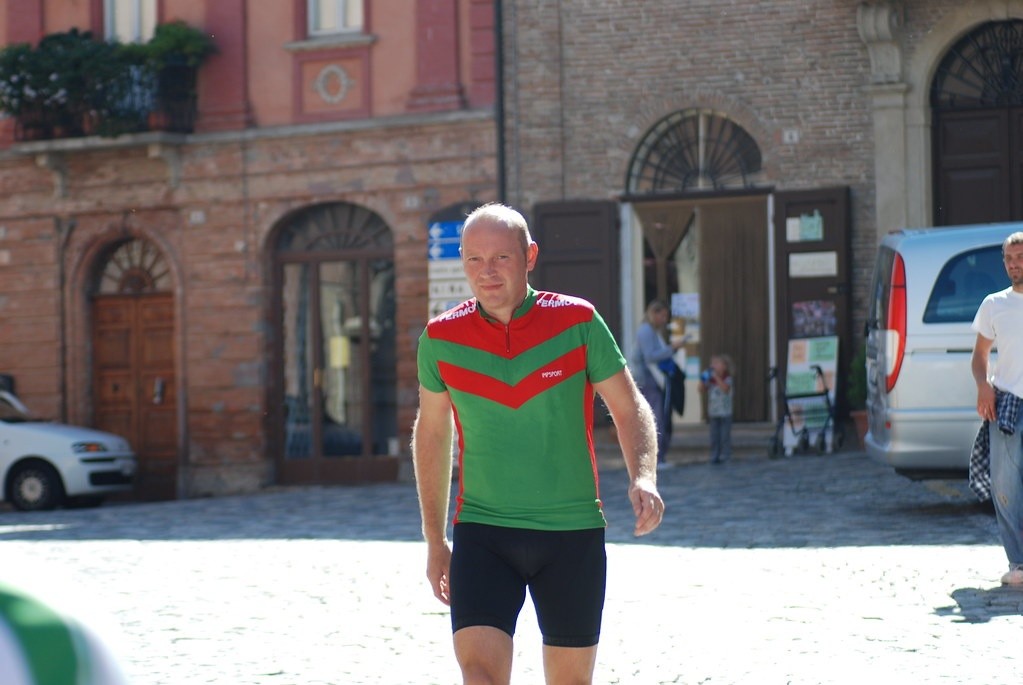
[281,396,379,459]
[0,391,138,514]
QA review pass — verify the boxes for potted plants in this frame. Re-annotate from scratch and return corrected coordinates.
[87,41,147,136]
[144,17,220,130]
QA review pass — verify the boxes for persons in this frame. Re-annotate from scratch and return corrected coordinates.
[632,300,686,473]
[698,354,735,466]
[969,232,1023,585]
[411,202,665,685]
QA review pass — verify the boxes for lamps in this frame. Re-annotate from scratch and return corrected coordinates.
[326,300,353,370]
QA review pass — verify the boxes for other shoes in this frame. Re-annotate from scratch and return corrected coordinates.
[656,460,675,470]
[1001,570,1023,585]
[711,453,731,464]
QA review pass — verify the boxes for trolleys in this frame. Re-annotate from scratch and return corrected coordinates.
[753,366,851,458]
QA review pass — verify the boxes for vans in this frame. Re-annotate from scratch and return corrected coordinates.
[861,222,1023,481]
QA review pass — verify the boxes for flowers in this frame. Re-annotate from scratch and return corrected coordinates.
[0,27,93,139]
